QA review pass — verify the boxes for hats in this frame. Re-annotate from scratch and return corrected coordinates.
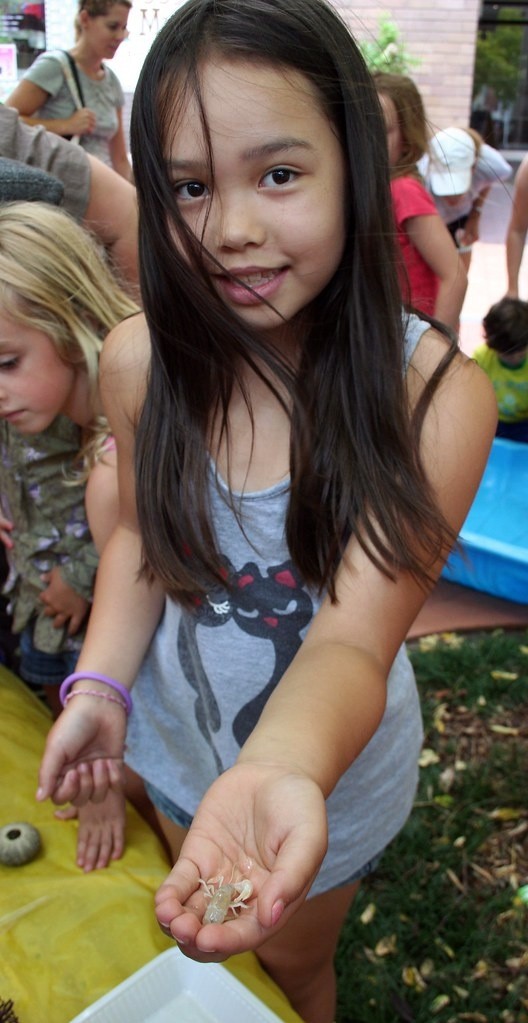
[430,126,475,197]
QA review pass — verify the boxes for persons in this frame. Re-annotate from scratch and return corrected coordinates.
[0,102,143,309]
[5,0,132,182]
[372,71,468,330]
[506,155,528,300]
[0,201,141,874]
[35,0,499,1023]
[415,127,514,273]
[471,298,528,446]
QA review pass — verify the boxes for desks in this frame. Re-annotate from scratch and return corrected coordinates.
[0,665,305,1023]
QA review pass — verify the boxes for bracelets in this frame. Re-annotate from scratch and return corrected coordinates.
[59,671,133,716]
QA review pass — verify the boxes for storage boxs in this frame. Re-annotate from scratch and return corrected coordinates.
[68,945,284,1023]
[440,437,528,608]
